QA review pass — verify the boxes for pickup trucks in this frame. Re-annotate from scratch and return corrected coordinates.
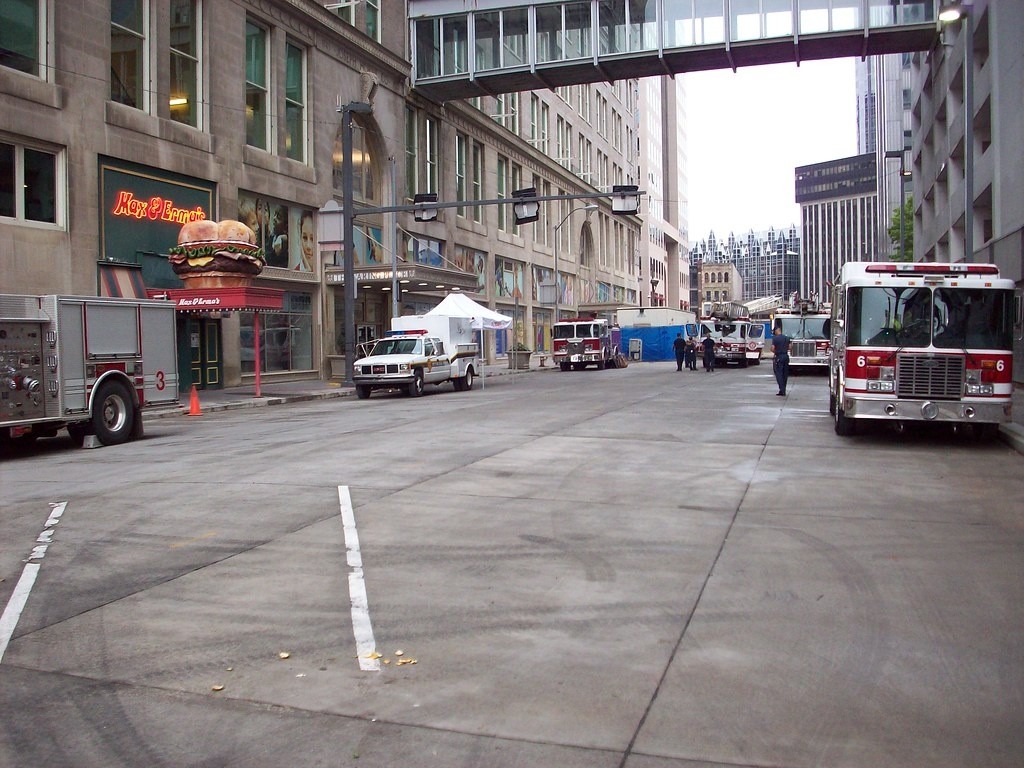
[352,313,479,399]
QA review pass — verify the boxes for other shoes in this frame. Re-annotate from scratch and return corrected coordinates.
[690,366,693,370]
[711,367,714,371]
[694,367,697,370]
[677,368,682,371]
[707,370,710,372]
[776,392,785,396]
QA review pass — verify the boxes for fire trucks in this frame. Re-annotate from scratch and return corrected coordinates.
[683,291,782,368]
[0,294,181,450]
[550,310,621,372]
[769,289,832,374]
[827,260,1024,446]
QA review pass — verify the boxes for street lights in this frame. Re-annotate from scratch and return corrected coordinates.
[939,0,975,267]
[554,204,598,324]
[883,150,913,261]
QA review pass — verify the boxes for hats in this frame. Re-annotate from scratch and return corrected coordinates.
[774,326,779,334]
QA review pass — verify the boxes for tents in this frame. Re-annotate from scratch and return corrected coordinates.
[425,287,515,390]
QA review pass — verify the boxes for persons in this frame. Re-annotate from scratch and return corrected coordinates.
[293,210,315,273]
[880,315,902,330]
[239,201,259,243]
[496,262,512,297]
[684,336,699,371]
[770,326,792,396]
[478,256,484,273]
[255,198,289,268]
[702,333,715,372]
[672,333,686,371]
[369,244,381,262]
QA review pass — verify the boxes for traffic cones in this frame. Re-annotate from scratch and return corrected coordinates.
[187,385,204,416]
